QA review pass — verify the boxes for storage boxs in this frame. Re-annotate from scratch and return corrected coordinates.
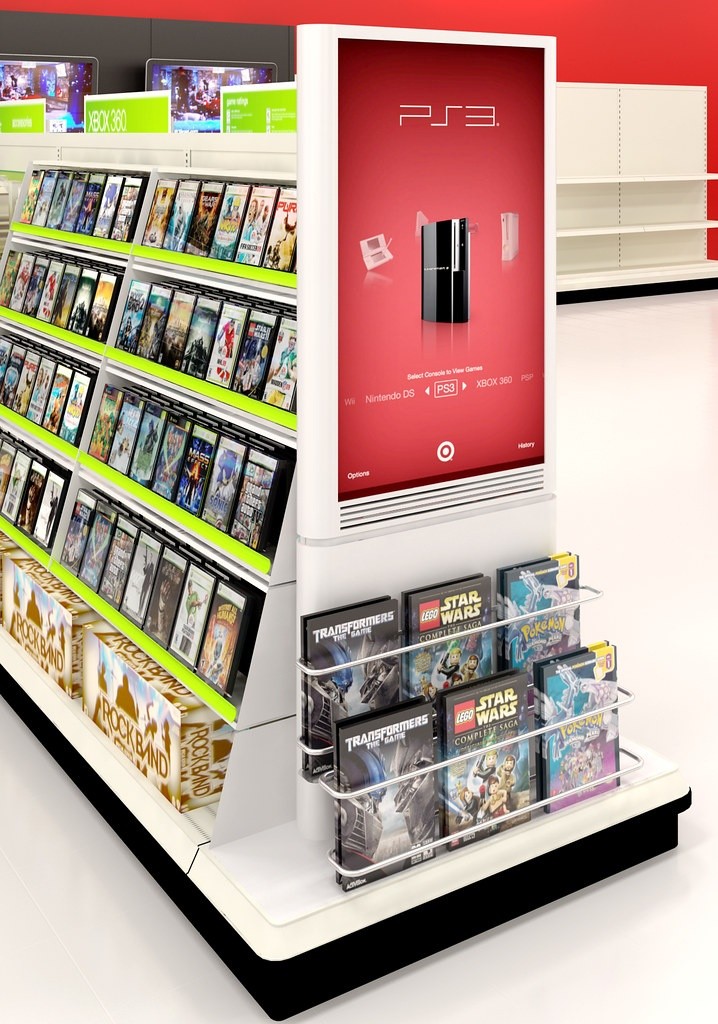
[0,530,235,813]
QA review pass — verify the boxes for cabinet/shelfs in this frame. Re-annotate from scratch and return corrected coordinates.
[556,172,718,304]
[0,159,297,890]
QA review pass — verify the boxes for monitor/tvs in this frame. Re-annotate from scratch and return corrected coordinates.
[145,58,277,132]
[0,54,99,134]
